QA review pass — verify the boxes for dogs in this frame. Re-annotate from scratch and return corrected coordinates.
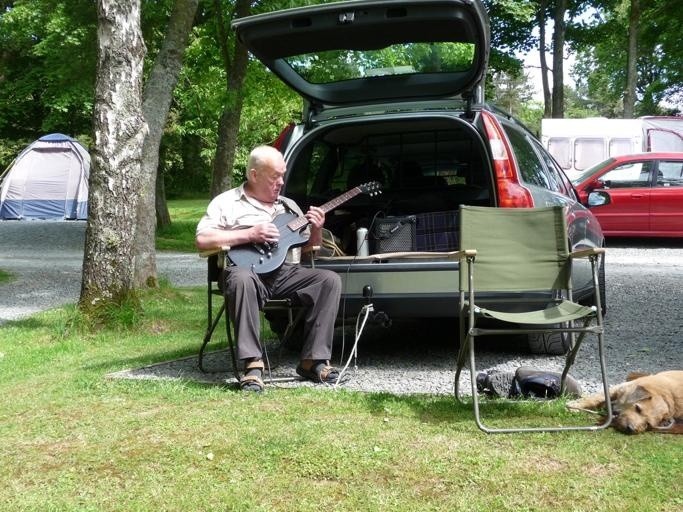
[563,368,683,437]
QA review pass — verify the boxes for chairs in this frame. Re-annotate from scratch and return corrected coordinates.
[195,239,344,383]
[346,156,427,195]
[452,200,617,434]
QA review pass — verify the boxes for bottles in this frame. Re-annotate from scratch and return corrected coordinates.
[355,227,370,258]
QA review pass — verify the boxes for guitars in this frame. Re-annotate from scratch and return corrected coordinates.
[227,181,384,274]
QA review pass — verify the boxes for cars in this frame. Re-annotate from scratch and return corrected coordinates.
[558,153,683,237]
[230,0,606,355]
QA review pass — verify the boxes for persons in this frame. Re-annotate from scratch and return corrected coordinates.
[194,145,353,392]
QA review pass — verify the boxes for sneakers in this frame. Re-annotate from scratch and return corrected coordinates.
[475,360,582,400]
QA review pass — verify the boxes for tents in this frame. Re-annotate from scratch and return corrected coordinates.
[1,132,91,220]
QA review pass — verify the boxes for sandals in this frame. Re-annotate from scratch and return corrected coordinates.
[296,359,348,384]
[239,359,264,393]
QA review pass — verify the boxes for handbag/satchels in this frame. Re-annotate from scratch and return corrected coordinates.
[300,227,348,258]
